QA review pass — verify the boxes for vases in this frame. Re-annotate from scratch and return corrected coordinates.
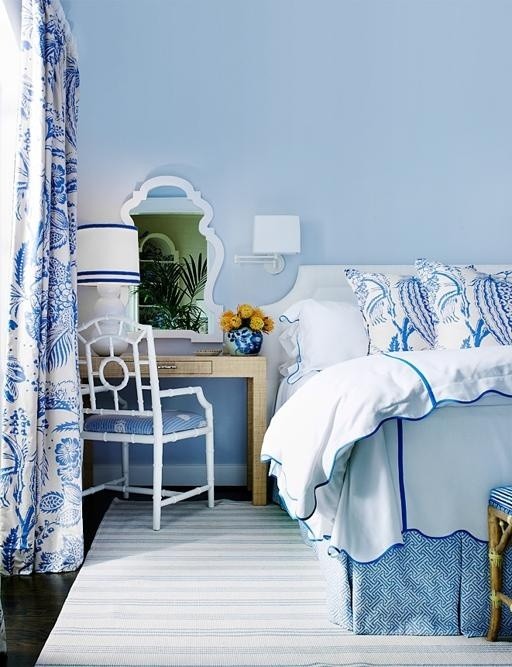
[229,327,263,355]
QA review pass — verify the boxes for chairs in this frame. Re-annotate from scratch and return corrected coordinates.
[73,316,215,531]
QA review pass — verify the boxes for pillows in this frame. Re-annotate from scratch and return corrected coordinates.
[279,262,512,384]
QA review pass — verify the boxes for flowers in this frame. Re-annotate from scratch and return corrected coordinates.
[220,304,275,335]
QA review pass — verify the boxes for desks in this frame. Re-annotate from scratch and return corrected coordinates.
[75,355,268,505]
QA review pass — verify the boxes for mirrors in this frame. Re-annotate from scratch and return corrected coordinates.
[119,176,225,343]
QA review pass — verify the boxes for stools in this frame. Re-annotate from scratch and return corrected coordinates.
[486,486,512,641]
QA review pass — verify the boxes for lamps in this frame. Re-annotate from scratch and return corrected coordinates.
[76,224,142,356]
[234,215,302,275]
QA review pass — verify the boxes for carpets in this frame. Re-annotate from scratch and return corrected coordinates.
[33,495,512,666]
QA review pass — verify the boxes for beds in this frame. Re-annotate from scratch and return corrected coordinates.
[264,266,512,635]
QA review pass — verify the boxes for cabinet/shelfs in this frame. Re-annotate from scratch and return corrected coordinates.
[139,233,179,309]
